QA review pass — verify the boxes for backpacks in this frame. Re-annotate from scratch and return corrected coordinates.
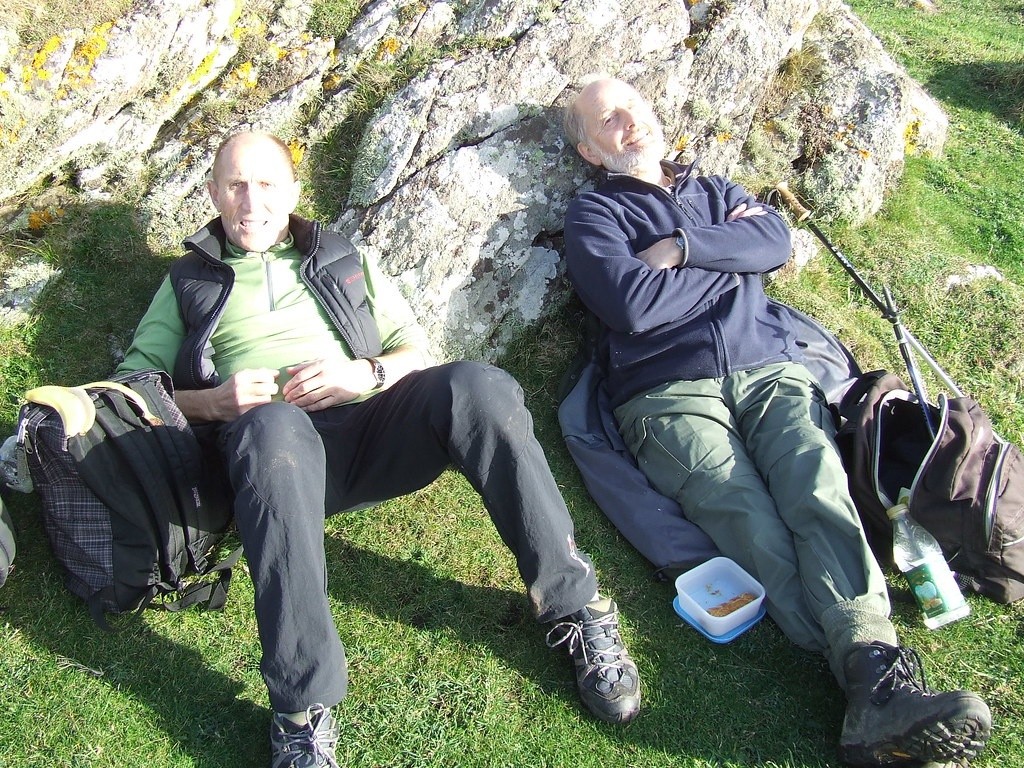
[16,367,244,633]
[834,368,1024,604]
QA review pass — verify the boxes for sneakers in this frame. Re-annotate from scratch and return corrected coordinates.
[270,703,340,768]
[553,595,641,725]
[838,640,992,768]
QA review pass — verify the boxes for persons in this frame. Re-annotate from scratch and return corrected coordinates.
[109,130,645,768]
[563,77,993,768]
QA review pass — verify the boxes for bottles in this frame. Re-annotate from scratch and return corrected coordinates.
[886,504,970,629]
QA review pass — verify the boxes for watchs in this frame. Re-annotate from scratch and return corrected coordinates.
[361,356,387,390]
[675,234,685,250]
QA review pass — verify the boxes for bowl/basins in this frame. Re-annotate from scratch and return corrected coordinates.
[675,557,766,637]
